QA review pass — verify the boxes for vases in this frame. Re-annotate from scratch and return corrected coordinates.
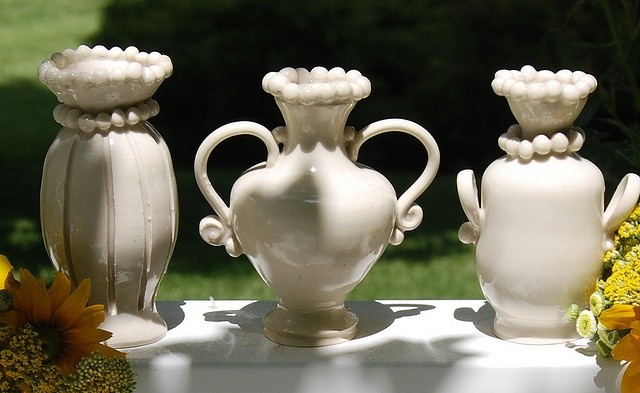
[194,67,441,348]
[39,44,178,349]
[456,65,640,346]
